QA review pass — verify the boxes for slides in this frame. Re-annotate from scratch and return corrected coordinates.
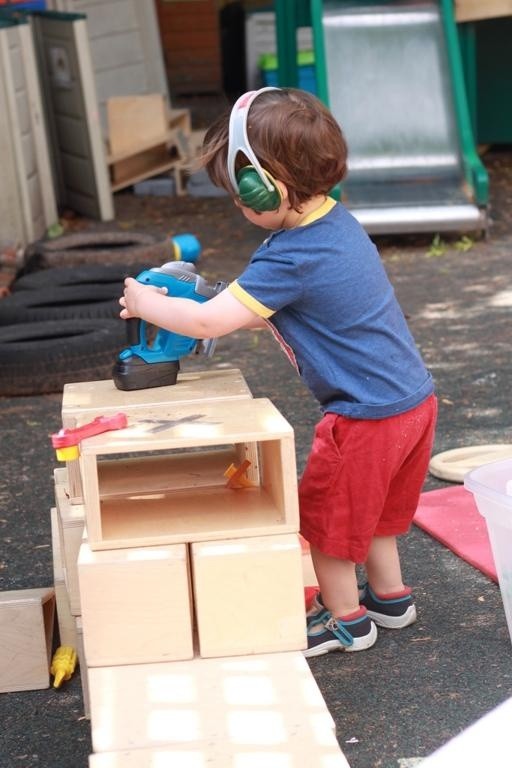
[311,0,489,242]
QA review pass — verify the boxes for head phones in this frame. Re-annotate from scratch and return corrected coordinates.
[223,87,284,216]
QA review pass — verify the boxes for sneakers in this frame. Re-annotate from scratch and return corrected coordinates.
[357,581,417,629]
[302,607,378,657]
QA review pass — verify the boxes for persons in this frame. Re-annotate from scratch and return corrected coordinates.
[118,87,438,658]
